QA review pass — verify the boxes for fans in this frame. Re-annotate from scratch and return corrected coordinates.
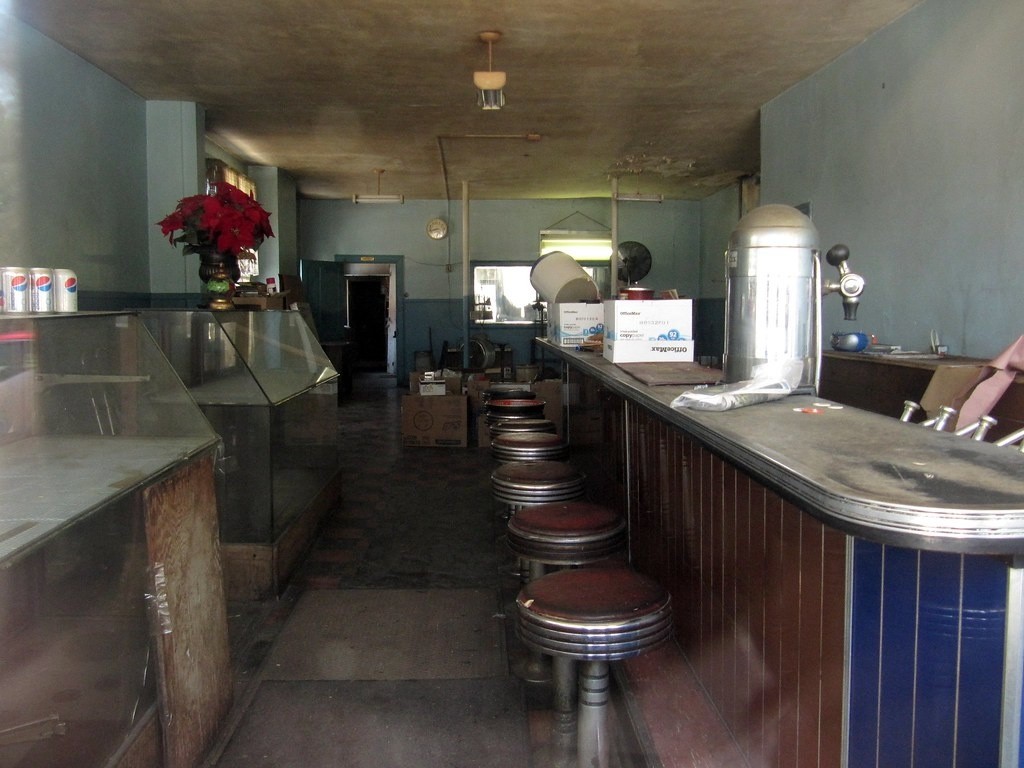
[609,241,652,287]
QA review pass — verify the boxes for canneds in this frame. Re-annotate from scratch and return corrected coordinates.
[0,266,78,315]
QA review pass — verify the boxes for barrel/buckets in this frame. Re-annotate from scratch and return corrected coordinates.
[529,250,599,303]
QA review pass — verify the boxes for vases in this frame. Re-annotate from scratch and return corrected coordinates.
[198,250,241,309]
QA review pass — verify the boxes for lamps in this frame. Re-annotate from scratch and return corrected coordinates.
[613,170,663,203]
[472,31,507,110]
[353,169,404,205]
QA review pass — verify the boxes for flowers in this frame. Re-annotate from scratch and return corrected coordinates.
[157,178,274,258]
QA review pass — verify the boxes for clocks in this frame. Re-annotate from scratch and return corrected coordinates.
[426,218,448,239]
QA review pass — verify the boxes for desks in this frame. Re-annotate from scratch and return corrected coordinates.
[321,341,357,393]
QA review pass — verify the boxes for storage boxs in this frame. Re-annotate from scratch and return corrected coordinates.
[545,302,603,347]
[401,372,564,446]
[601,296,695,364]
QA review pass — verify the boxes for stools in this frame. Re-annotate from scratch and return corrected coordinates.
[484,411,545,425]
[506,502,626,737]
[497,432,567,511]
[481,388,537,400]
[489,418,557,521]
[515,568,674,768]
[492,460,584,581]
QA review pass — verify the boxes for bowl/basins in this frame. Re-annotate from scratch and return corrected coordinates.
[617,286,655,299]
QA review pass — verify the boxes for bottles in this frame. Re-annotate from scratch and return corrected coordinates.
[266,278,277,294]
